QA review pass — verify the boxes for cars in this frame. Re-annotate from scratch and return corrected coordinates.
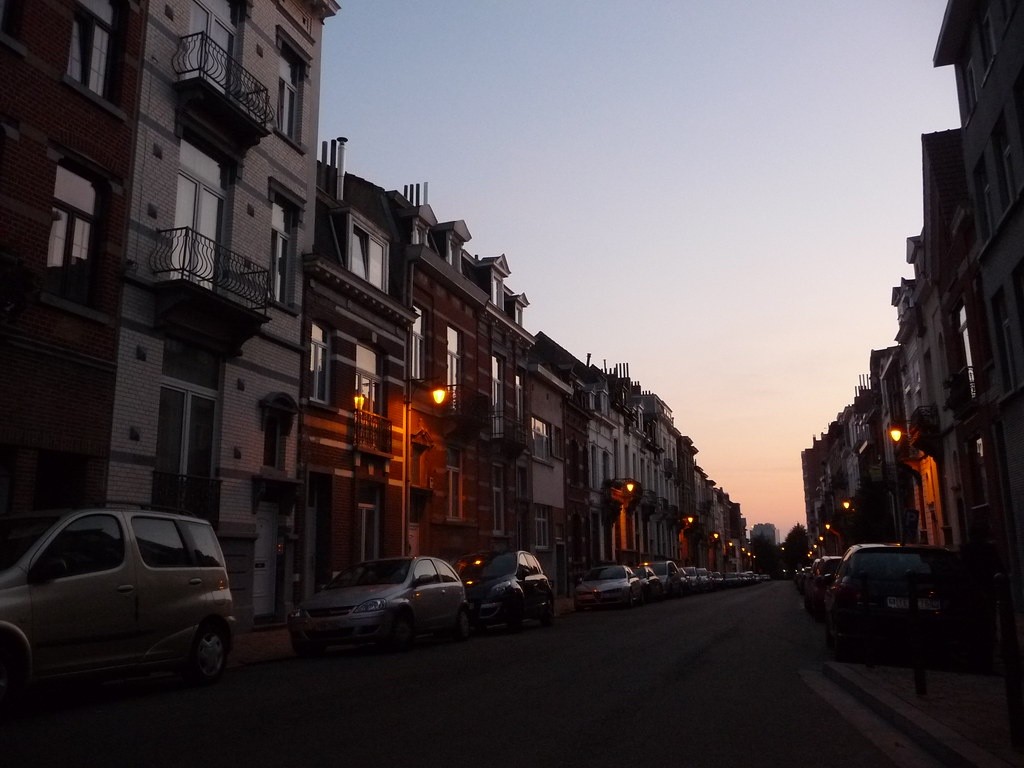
[792,556,843,622]
[629,566,663,602]
[287,555,472,654]
[574,564,643,612]
[677,566,770,591]
[638,561,682,599]
[449,550,555,631]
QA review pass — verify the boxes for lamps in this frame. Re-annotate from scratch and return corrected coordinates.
[402,375,450,406]
[355,381,377,410]
[890,418,911,441]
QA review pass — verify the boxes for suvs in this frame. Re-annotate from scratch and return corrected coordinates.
[0,501,236,699]
[822,543,958,658]
